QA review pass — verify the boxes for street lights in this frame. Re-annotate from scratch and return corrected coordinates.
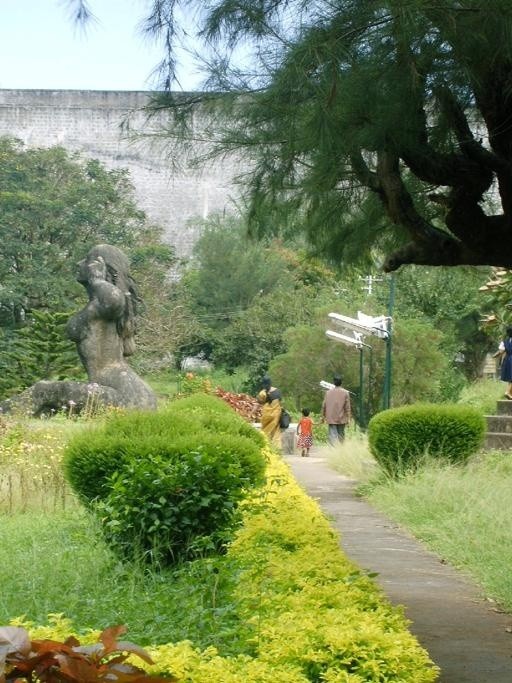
[319,378,358,436]
[323,329,375,435]
[330,309,392,417]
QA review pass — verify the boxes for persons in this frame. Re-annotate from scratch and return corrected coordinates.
[320,375,351,447]
[493,326,512,400]
[296,407,324,457]
[0,243,158,416]
[257,376,282,450]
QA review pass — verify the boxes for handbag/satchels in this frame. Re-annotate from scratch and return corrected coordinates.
[279,408,291,428]
